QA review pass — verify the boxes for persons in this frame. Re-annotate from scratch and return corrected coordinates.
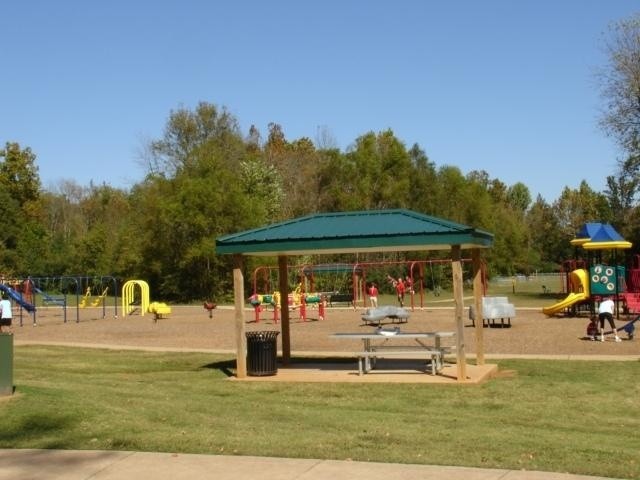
[598,298,622,342]
[396,278,405,307]
[405,276,411,293]
[24,280,32,304]
[369,282,378,307]
[0,295,13,333]
[587,316,597,341]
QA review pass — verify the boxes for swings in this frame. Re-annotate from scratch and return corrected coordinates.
[429,262,444,296]
[368,264,415,292]
[1,279,104,321]
[267,265,349,297]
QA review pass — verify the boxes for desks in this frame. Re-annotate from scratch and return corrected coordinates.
[327,328,457,369]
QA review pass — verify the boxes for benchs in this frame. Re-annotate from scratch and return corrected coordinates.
[354,345,453,375]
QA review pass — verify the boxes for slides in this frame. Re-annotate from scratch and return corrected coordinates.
[542,293,587,316]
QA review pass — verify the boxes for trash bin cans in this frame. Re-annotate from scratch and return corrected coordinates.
[246,331,279,375]
[0,333,13,394]
